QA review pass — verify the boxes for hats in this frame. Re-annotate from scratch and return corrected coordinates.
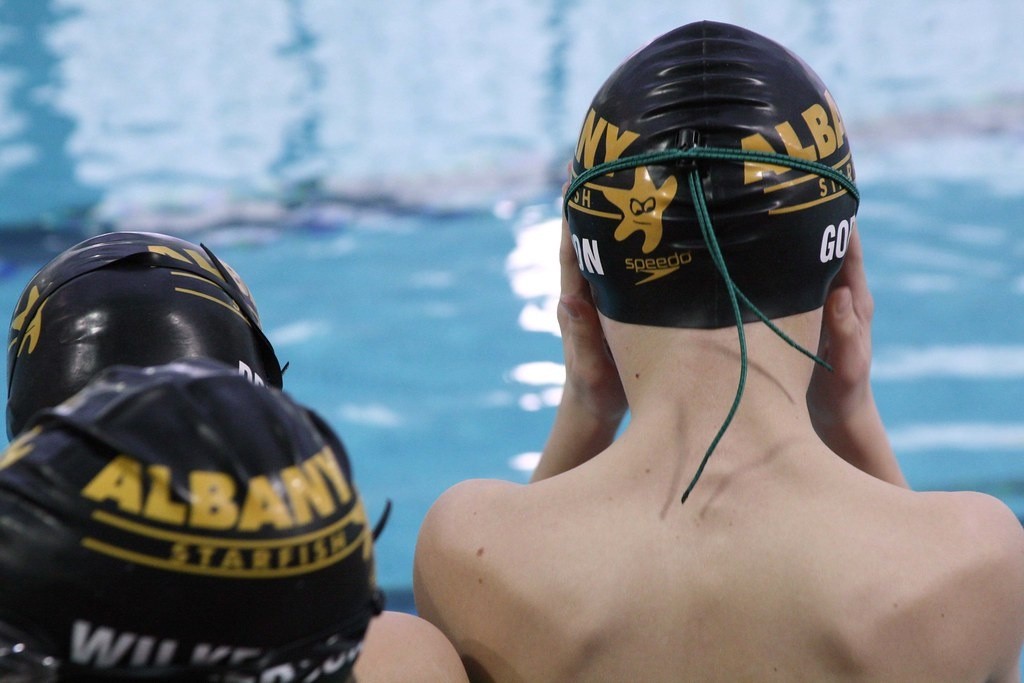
[565,20,859,330]
[0,230,391,683]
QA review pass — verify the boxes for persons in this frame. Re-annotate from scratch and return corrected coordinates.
[0,230,471,683]
[411,20,1024,683]
